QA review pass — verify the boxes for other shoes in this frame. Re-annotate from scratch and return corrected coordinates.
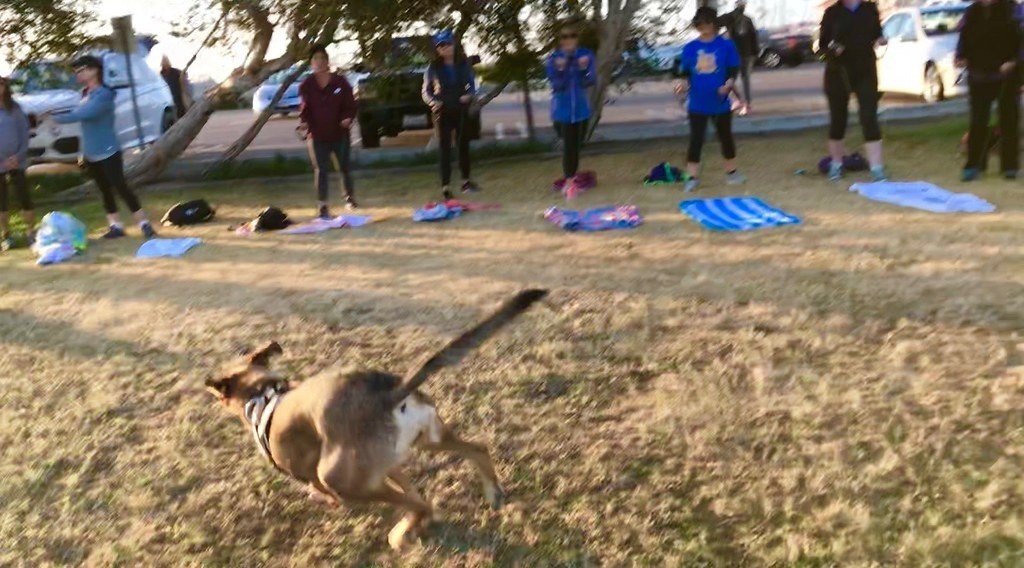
[731,100,742,111]
[684,178,700,191]
[739,104,751,116]
[871,168,887,182]
[345,196,359,211]
[25,236,36,246]
[1004,170,1016,179]
[461,181,483,193]
[318,205,329,220]
[828,165,846,183]
[959,169,978,181]
[562,179,586,201]
[441,190,453,200]
[1,236,17,251]
[725,172,747,185]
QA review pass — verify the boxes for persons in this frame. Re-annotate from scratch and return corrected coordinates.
[819,0,889,182]
[954,0,1024,182]
[672,6,740,193]
[548,25,597,189]
[421,32,476,200]
[294,46,357,219]
[160,54,184,117]
[43,56,155,240]
[718,0,760,119]
[0,77,35,251]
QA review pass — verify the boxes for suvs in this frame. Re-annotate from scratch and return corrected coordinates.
[351,35,481,148]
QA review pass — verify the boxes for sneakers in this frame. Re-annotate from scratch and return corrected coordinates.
[100,225,125,239]
[141,222,157,240]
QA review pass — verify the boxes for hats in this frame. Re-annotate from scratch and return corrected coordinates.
[68,56,101,67]
[435,31,456,47]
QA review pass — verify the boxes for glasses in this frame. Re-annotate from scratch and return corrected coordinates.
[694,18,713,26]
[560,33,577,39]
[438,42,452,47]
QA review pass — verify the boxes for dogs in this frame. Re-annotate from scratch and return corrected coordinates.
[204,281,551,544]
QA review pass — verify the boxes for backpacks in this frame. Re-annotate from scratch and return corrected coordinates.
[249,207,291,232]
[818,152,869,174]
[160,198,215,227]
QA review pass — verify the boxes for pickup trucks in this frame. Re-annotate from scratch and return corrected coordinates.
[755,28,813,70]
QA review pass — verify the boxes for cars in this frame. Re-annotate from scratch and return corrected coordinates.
[253,68,313,116]
[6,48,178,165]
[873,4,970,104]
